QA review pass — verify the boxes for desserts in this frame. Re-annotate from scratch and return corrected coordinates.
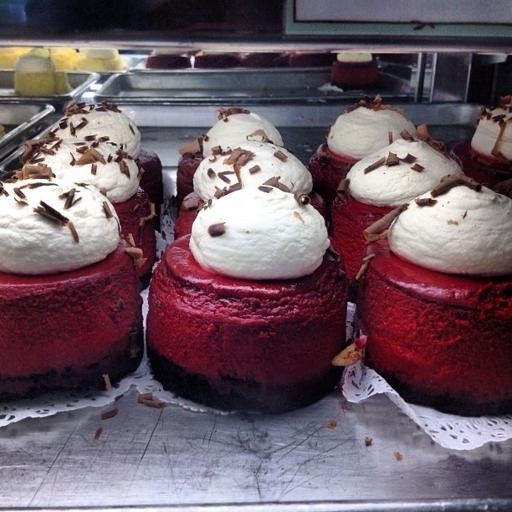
[0,98,512,416]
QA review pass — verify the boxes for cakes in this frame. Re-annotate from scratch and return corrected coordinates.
[146,46,380,92]
[0,45,126,99]
